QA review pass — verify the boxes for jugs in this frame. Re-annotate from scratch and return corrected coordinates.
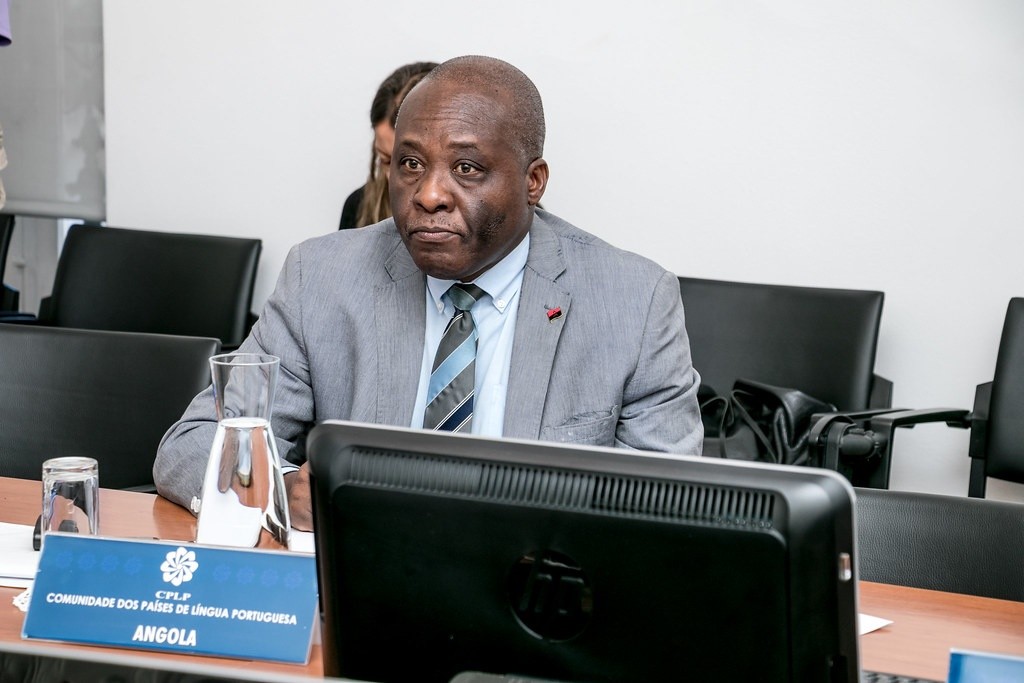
[196,353,291,551]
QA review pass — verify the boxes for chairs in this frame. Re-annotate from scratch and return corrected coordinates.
[666,274,910,486]
[0,208,266,499]
[869,290,1024,507]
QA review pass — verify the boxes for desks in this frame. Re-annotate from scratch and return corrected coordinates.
[0,478,329,683]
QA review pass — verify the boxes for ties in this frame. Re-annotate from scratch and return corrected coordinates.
[422,282,485,433]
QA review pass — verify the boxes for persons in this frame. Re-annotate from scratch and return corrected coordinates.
[340,61,440,231]
[153,54,707,531]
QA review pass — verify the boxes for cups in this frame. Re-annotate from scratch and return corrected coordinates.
[40,457,99,537]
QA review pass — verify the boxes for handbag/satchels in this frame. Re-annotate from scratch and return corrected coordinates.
[696,377,844,466]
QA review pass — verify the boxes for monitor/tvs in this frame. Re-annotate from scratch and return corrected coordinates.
[306,418,861,682]
